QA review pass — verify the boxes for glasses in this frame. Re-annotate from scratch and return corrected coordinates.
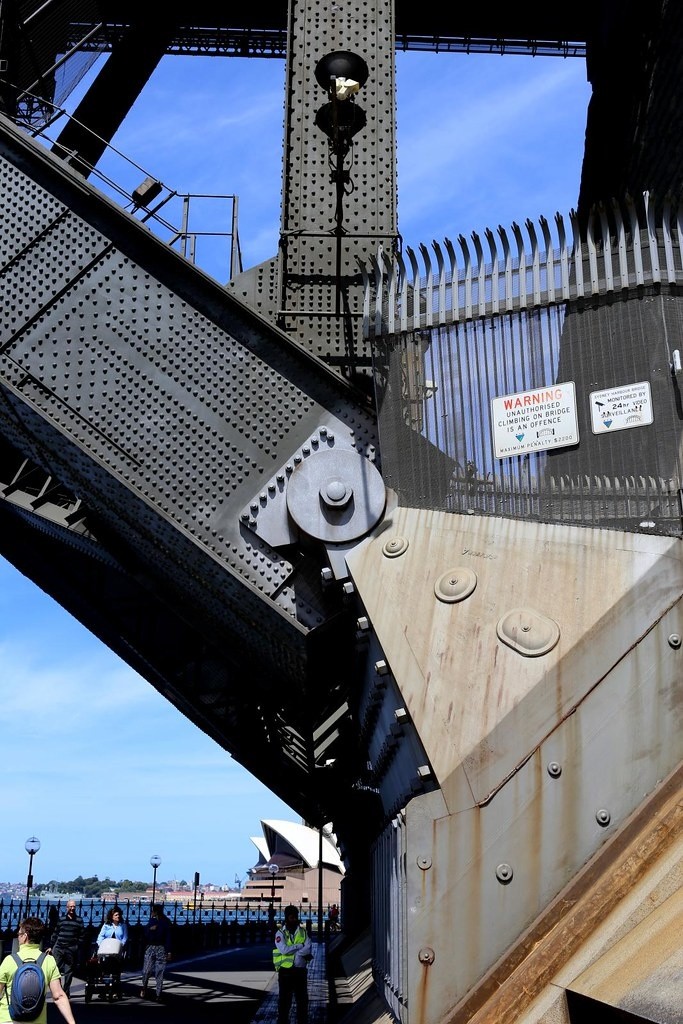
[17,932,23,935]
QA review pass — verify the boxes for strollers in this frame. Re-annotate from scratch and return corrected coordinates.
[83,937,126,1003]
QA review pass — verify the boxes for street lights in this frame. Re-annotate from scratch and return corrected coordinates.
[149,854,162,905]
[25,836,41,924]
[266,862,281,922]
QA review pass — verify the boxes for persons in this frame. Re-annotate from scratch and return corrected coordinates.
[96,907,128,1001]
[45,900,85,999]
[139,904,171,1001]
[0,917,76,1024]
[272,906,312,1024]
[328,904,340,931]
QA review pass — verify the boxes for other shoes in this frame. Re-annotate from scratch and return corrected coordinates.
[141,989,146,998]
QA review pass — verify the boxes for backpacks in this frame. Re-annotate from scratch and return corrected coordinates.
[4,951,47,1022]
